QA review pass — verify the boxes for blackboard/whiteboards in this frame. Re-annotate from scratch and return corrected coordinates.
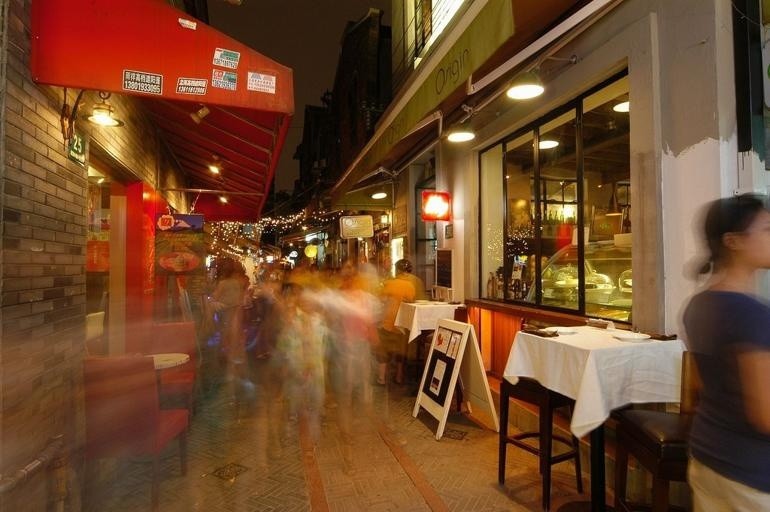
[435,249,453,290]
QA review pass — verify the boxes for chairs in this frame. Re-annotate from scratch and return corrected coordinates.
[495,312,587,511]
[613,350,703,512]
[80,352,196,511]
[145,322,201,419]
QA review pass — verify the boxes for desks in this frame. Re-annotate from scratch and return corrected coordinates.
[395,298,468,399]
[501,324,688,512]
[140,352,192,411]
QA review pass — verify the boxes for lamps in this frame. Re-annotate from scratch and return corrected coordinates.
[371,179,402,200]
[190,103,209,123]
[60,86,127,141]
[446,107,503,143]
[503,49,580,100]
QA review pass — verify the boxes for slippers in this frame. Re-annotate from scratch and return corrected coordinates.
[369,375,386,387]
[391,374,405,385]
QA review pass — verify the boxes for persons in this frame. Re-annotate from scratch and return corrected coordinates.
[680,193,768,511]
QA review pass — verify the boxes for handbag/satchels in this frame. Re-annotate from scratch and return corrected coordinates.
[197,311,216,337]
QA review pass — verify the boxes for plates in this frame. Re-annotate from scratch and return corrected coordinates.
[407,298,448,306]
[574,284,617,296]
[554,278,591,289]
[611,330,651,344]
[544,325,583,336]
